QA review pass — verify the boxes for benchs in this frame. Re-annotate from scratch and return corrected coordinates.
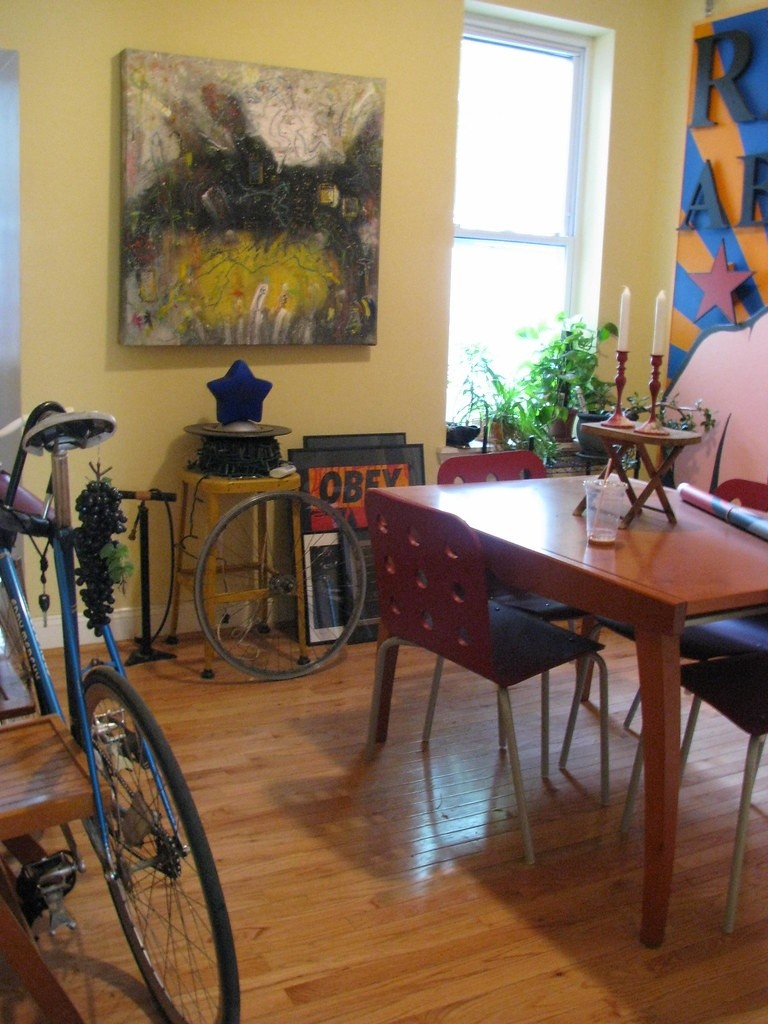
[0,708,113,1024]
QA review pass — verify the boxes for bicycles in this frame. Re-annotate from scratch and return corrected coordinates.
[0,401,243,1024]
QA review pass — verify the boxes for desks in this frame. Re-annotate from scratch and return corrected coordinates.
[164,469,310,678]
[363,473,768,944]
[572,423,701,530]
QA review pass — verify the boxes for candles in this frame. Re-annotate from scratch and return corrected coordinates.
[650,290,667,354]
[619,285,630,353]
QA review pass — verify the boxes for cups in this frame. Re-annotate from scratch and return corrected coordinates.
[583,478,628,546]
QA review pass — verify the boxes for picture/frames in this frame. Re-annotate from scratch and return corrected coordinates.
[301,528,376,646]
[286,443,425,647]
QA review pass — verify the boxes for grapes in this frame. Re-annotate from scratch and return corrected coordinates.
[76,482,128,638]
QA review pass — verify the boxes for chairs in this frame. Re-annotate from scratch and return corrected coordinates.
[366,484,610,866]
[559,478,768,786]
[421,450,589,777]
[618,652,768,935]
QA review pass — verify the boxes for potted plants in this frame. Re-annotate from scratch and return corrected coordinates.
[453,310,716,474]
